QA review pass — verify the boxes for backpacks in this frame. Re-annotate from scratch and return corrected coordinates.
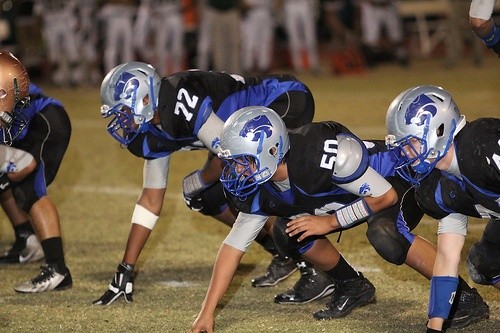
[328,39,365,76]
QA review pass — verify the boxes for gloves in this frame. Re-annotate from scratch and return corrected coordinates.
[182,192,207,211]
[92,264,134,305]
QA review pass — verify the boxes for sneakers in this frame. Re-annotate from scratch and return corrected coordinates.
[313,271,376,319]
[0,233,45,263]
[426,288,490,329]
[274,264,336,304]
[13,264,72,292]
[251,253,301,287]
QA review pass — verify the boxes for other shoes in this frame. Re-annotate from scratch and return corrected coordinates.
[392,39,411,65]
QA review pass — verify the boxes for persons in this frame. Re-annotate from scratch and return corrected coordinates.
[92,62,316,308]
[0,49,74,294]
[468,0,500,58]
[0,0,411,92]
[186,105,492,333]
[384,85,500,333]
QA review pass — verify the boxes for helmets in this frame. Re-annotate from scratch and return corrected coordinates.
[385,84,461,190]
[0,51,31,147]
[219,105,291,202]
[99,61,161,146]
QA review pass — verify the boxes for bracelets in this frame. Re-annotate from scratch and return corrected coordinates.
[131,203,159,231]
[335,197,372,229]
[427,275,460,320]
